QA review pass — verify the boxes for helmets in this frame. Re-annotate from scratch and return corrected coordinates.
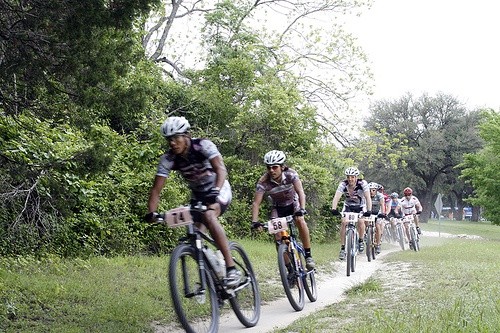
[161,116,191,137]
[345,167,359,176]
[263,149,286,164]
[391,192,399,198]
[404,187,413,193]
[378,184,384,189]
[368,182,378,189]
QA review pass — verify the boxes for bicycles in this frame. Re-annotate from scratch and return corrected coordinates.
[398,213,421,252]
[154,198,261,333]
[259,210,318,312]
[337,209,366,276]
[361,212,409,262]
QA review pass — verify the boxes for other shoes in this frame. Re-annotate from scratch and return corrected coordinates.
[416,227,421,234]
[338,250,345,259]
[377,245,381,253]
[409,240,414,250]
[358,239,364,253]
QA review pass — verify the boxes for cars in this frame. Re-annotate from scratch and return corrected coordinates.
[431,206,473,220]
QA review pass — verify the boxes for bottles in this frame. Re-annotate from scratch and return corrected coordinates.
[218,247,227,278]
[202,245,222,274]
[293,248,300,260]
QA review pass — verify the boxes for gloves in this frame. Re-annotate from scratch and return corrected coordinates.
[201,187,220,206]
[331,209,340,216]
[144,212,160,223]
[364,211,372,217]
[378,214,386,217]
[416,211,422,214]
[397,216,402,219]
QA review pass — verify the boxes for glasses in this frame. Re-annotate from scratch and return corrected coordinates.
[370,190,376,192]
[267,165,279,170]
[404,195,410,196]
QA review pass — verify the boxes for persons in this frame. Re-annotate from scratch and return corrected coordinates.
[394,188,423,250]
[367,182,408,254]
[251,150,314,288]
[331,167,372,259]
[145,117,244,286]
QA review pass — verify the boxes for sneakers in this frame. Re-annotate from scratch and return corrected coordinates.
[222,270,246,286]
[306,257,315,266]
[288,268,296,288]
